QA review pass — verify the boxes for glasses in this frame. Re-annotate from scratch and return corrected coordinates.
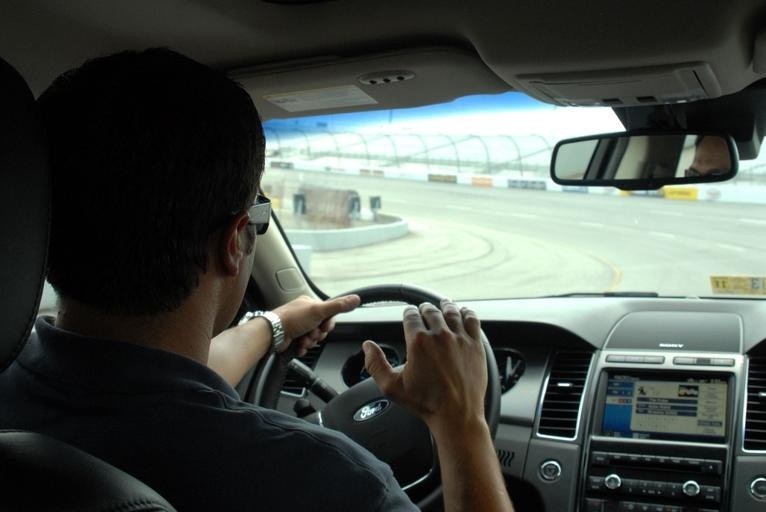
[244,193,271,234]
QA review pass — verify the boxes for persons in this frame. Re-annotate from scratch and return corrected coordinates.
[0,49,517,511]
[682,136,733,179]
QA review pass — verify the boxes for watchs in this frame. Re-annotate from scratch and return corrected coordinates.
[238,310,287,355]
